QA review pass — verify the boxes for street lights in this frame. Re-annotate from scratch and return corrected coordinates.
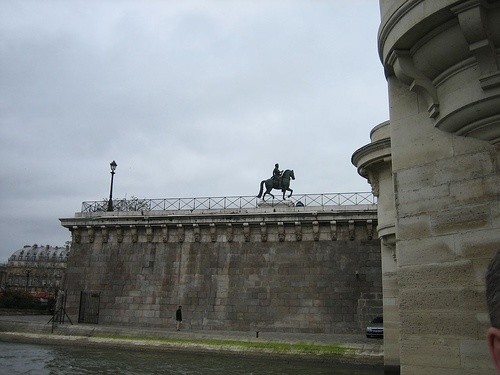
[106,160,118,212]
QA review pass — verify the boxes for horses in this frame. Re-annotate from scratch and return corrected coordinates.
[255,169,296,203]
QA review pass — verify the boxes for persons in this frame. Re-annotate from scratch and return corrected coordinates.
[175,306,182,331]
[486,248,500,375]
[273,163,284,188]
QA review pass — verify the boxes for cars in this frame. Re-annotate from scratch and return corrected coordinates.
[365,315,383,339]
